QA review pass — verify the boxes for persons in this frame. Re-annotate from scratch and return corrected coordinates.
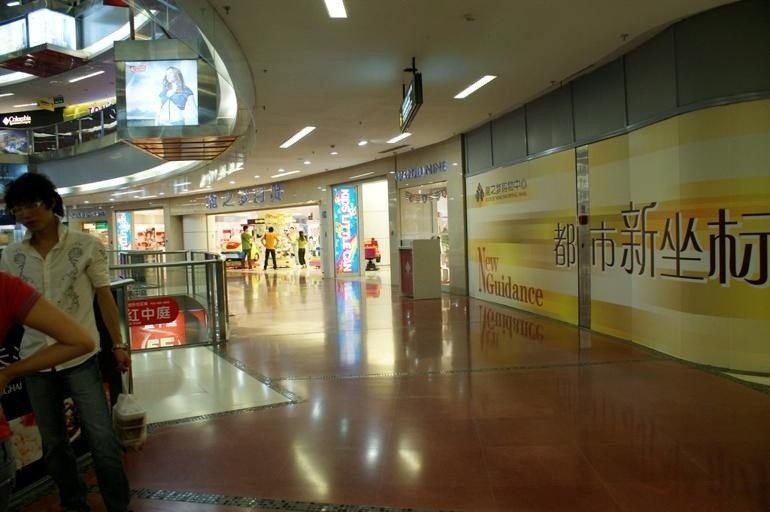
[241,225,279,270]
[156,66,198,126]
[287,227,308,269]
[0,171,132,512]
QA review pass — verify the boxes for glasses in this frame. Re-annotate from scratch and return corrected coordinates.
[10,200,43,215]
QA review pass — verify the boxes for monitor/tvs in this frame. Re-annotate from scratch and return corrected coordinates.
[125,60,199,126]
